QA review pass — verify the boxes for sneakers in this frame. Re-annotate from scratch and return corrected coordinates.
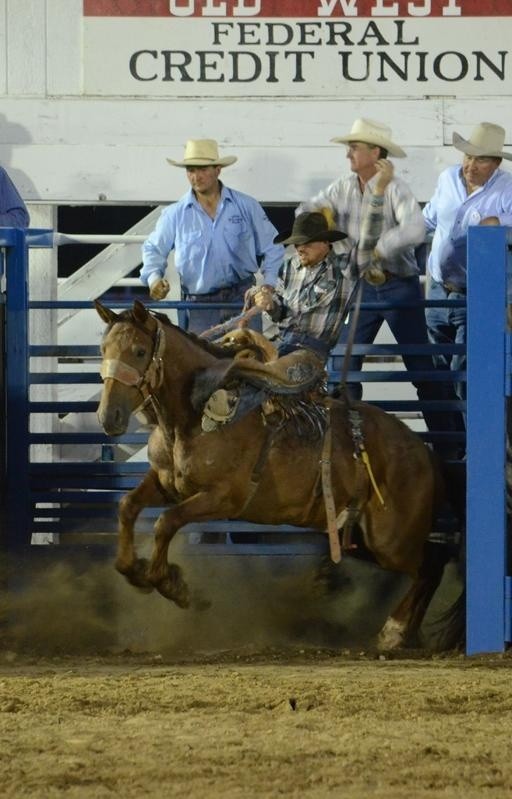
[201,388,231,430]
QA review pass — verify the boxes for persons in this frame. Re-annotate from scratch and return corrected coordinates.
[202,158,394,430]
[142,139,285,345]
[295,119,464,462]
[421,123,512,400]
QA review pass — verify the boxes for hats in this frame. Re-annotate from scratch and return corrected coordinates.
[452,123,511,160]
[272,212,347,245]
[168,140,238,167]
[330,120,407,159]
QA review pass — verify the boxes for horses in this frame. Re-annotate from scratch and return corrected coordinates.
[93,297,512,653]
